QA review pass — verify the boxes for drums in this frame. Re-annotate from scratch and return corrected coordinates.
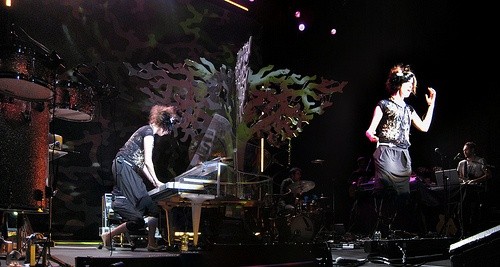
[288,214,316,242]
[52,78,95,123]
[298,202,317,214]
[1,41,57,102]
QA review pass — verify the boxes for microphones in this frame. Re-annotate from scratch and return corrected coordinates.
[453,153,460,160]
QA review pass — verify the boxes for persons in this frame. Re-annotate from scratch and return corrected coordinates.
[100,104,181,252]
[456,142,492,182]
[348,157,371,183]
[365,64,437,238]
[279,168,304,209]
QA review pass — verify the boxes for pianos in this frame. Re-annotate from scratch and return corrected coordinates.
[145,114,273,249]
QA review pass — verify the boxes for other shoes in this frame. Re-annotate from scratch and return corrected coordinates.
[146,244,167,252]
[101,233,111,251]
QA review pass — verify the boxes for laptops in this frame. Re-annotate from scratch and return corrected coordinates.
[435,169,460,187]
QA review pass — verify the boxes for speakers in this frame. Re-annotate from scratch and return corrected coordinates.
[449,225,500,267]
